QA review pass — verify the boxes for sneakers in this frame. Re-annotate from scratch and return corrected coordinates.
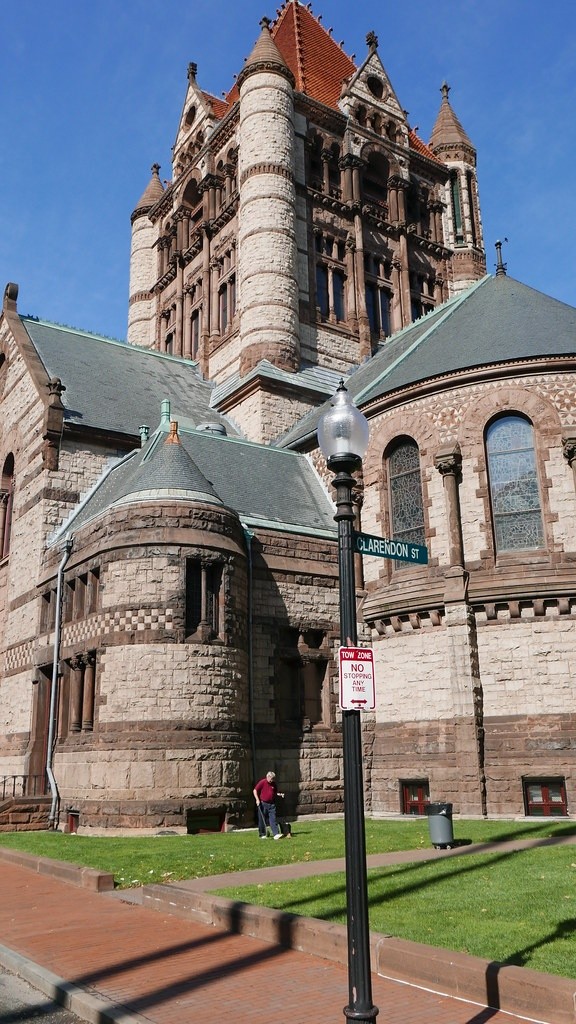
[273,833,284,840]
[260,836,267,840]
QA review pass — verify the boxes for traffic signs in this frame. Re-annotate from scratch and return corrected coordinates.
[353,530,428,565]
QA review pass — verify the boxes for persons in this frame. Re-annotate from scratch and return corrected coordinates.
[253,771,285,840]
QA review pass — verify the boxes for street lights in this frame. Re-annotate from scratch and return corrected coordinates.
[317,381,379,1024]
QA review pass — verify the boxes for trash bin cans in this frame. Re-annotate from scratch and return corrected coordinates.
[425,800,456,851]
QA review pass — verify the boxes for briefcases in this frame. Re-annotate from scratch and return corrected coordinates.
[278,822,291,834]
